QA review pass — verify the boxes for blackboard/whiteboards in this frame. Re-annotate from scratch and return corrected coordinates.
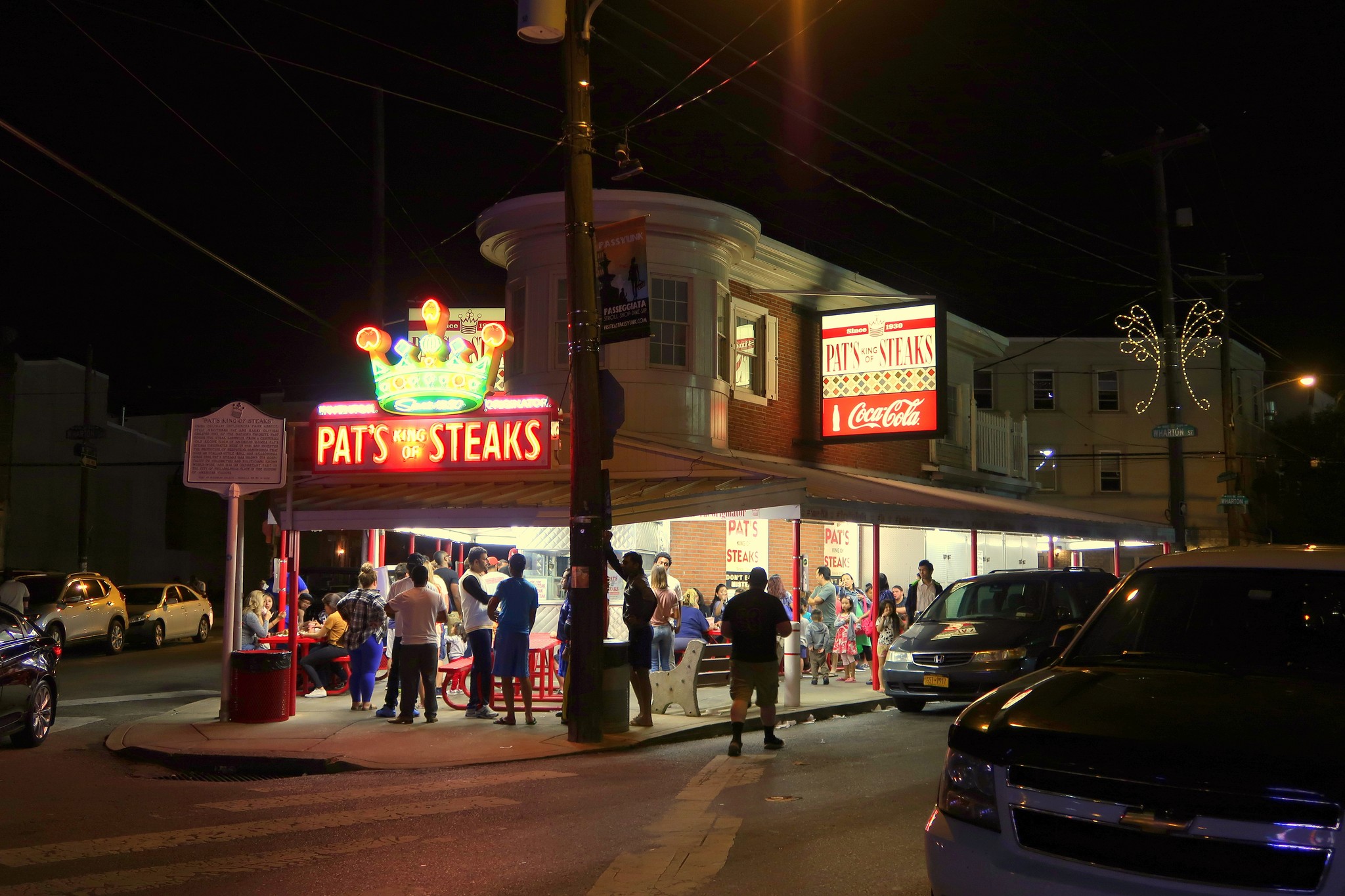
[523,575,552,600]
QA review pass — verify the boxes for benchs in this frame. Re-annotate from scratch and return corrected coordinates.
[438,656,474,705]
[674,650,685,666]
[649,636,784,717]
[332,646,389,692]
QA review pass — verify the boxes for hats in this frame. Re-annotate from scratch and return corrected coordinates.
[299,593,315,604]
[407,552,426,566]
[0,569,21,577]
[508,548,518,561]
[487,557,501,567]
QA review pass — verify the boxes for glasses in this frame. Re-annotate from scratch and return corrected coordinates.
[300,598,312,606]
[444,555,452,558]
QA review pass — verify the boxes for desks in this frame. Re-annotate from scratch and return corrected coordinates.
[257,635,326,696]
[700,630,729,671]
[488,632,561,711]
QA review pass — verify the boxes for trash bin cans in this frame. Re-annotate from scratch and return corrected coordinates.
[230,649,292,724]
[602,637,631,734]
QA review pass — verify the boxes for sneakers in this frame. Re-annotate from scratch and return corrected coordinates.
[412,686,465,716]
[475,704,499,719]
[304,688,327,698]
[376,704,396,717]
[552,689,564,695]
[800,663,885,693]
[465,704,476,717]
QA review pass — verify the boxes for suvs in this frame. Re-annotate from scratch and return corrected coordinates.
[921,544,1345,896]
[0,569,129,655]
[881,567,1121,712]
[299,565,359,622]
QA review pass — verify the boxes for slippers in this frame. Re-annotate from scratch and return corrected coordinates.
[351,702,363,710]
[525,716,537,724]
[361,703,377,711]
[630,716,653,727]
[493,717,516,725]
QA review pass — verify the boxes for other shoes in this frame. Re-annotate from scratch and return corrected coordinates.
[763,734,784,748]
[335,682,347,690]
[728,742,743,757]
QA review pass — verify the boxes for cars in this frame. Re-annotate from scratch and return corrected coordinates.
[116,584,213,650]
[0,601,61,749]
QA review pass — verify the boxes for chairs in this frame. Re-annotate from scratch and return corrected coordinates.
[1007,594,1024,610]
[980,599,999,616]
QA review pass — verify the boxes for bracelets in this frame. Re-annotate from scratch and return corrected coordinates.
[712,625,715,630]
[271,622,275,626]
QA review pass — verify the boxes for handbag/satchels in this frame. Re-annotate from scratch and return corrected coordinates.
[853,596,873,638]
[336,631,346,647]
[670,625,676,633]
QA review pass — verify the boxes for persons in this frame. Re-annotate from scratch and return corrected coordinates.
[458,546,540,725]
[801,566,895,684]
[549,567,571,716]
[375,550,471,724]
[171,574,206,598]
[0,569,29,615]
[242,572,347,697]
[647,552,683,672]
[876,600,909,693]
[768,574,793,621]
[892,586,907,620]
[905,560,943,628]
[674,588,718,651]
[602,531,657,726]
[693,588,708,618]
[709,584,747,628]
[720,567,793,756]
[336,563,388,710]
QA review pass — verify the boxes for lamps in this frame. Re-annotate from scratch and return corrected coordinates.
[1055,549,1061,556]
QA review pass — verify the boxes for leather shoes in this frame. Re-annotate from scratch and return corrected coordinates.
[427,717,438,723]
[388,712,413,724]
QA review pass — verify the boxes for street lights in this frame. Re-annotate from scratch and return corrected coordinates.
[1220,371,1318,545]
